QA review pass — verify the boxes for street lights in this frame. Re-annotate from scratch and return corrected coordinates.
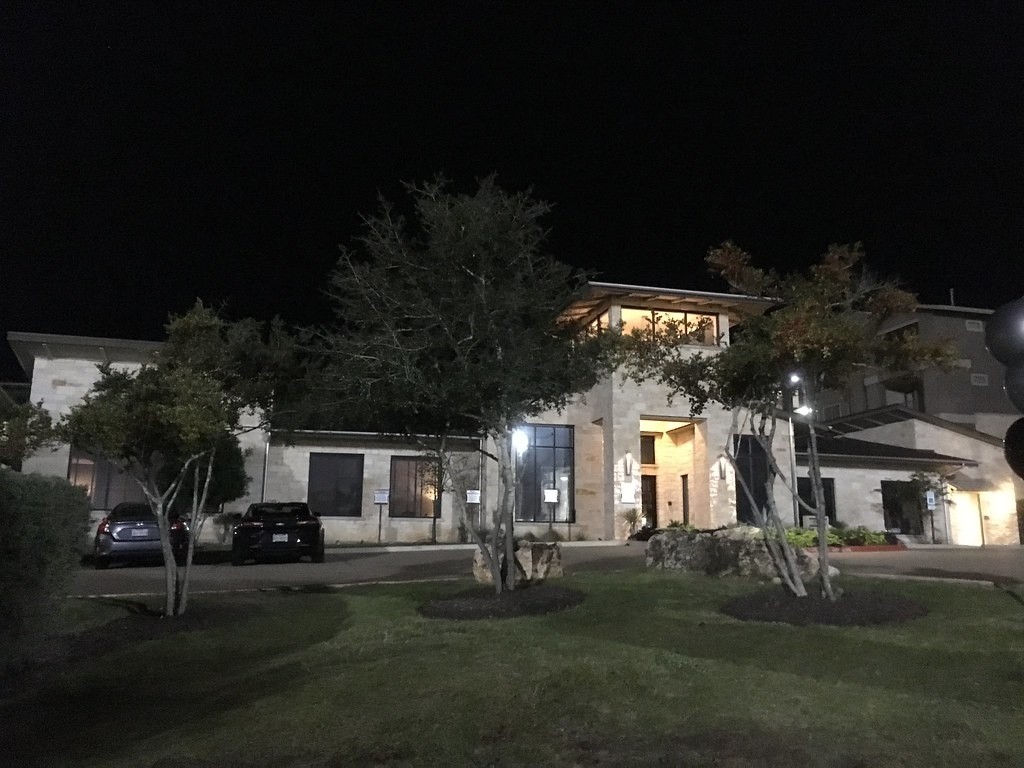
[785,375,800,527]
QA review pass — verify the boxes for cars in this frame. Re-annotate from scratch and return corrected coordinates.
[95,502,190,570]
[231,500,324,566]
